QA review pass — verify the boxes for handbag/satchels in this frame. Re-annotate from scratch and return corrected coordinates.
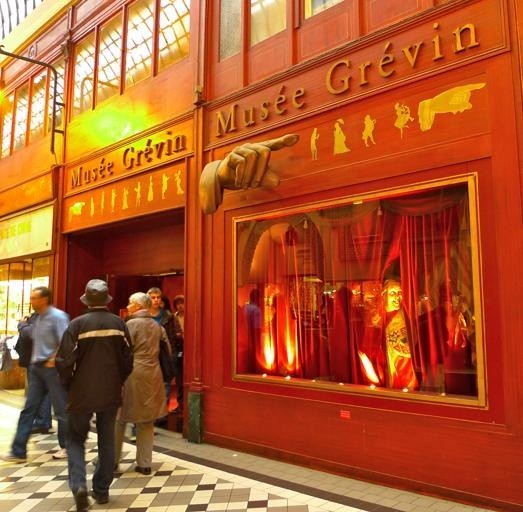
[158,340,174,383]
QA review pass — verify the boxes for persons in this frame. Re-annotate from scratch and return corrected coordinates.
[0,288,73,464]
[243,273,474,394]
[123,285,178,443]
[98,291,168,479]
[167,293,185,415]
[56,279,133,510]
[161,298,174,311]
[29,396,54,437]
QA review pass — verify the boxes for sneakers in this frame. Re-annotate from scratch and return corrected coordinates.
[73,487,109,512]
[0,451,27,463]
[135,466,151,475]
[52,447,69,459]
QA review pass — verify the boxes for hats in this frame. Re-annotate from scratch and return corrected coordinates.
[80,278,113,306]
